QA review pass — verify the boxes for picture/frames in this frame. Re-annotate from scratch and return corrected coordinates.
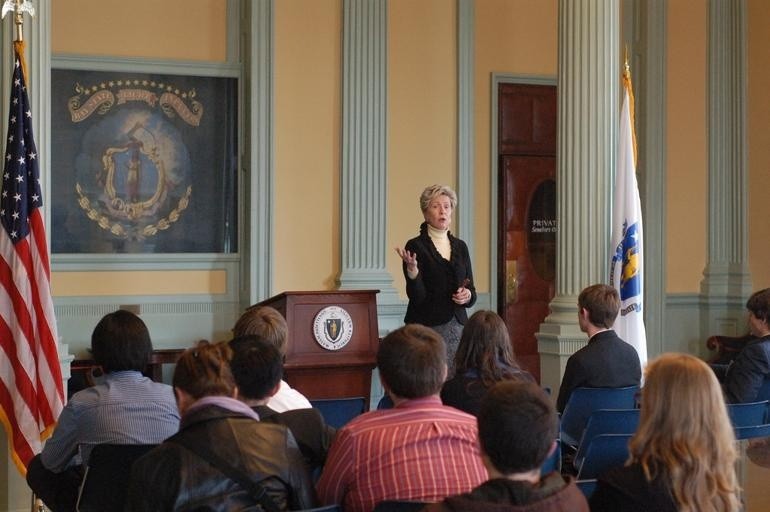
[50,53,241,270]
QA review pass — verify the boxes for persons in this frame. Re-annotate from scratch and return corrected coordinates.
[556,284,642,458]
[440,310,538,417]
[125,340,320,512]
[24,309,181,512]
[709,287,770,402]
[588,352,746,512]
[316,324,490,512]
[233,306,314,413]
[225,333,330,475]
[421,379,592,512]
[394,184,478,381]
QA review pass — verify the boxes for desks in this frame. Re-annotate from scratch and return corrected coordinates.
[72,350,185,382]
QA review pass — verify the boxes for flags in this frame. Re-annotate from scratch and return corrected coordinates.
[611,59,648,388]
[0,39,67,474]
